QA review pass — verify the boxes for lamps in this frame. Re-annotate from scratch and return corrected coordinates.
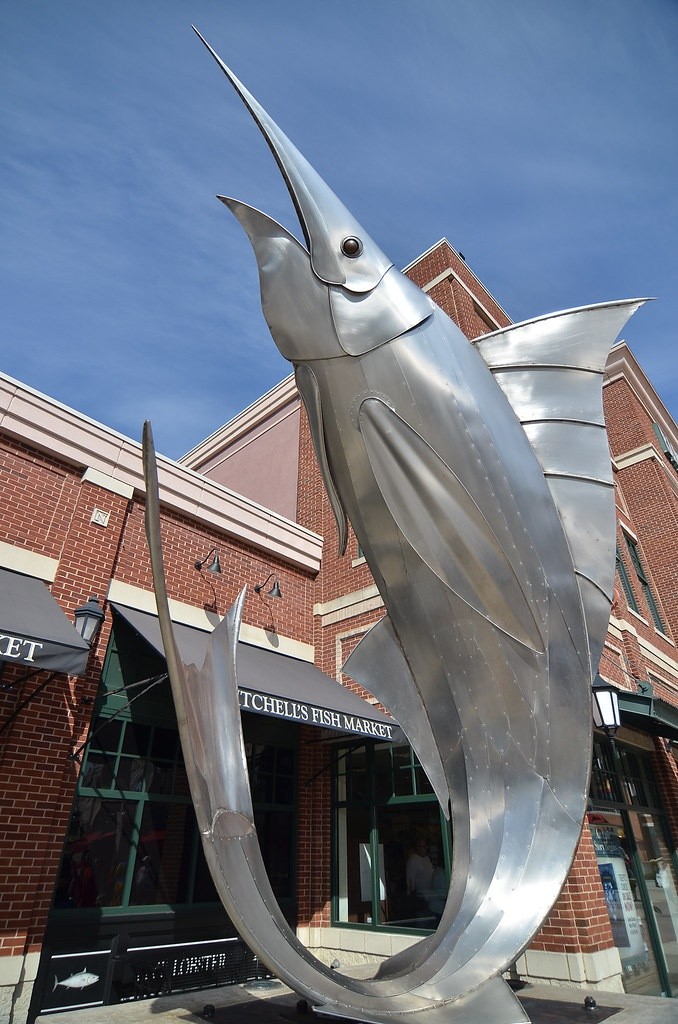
[194,546,222,574]
[254,572,283,599]
[591,669,622,737]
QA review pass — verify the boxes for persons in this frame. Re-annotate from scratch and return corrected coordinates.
[604,835,642,901]
[63,843,154,907]
[430,855,449,923]
[406,837,434,928]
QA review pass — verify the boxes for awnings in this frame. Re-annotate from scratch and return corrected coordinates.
[73,600,407,789]
[0,564,92,736]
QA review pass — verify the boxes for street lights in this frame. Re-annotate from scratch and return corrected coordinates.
[590,669,673,999]
